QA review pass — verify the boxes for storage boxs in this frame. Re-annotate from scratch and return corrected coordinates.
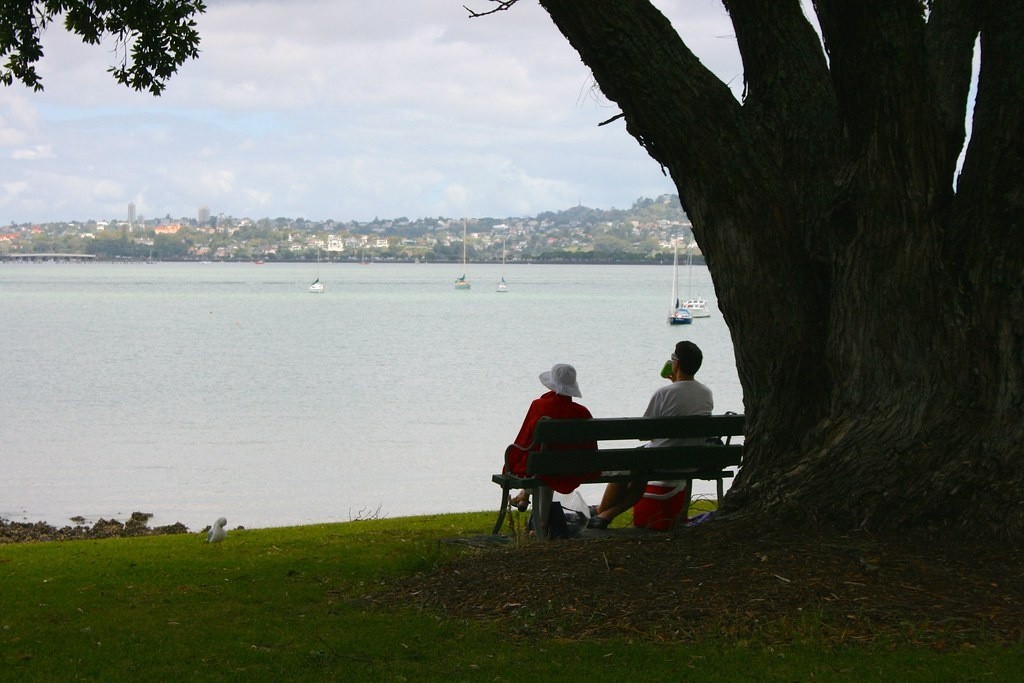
[634,478,689,530]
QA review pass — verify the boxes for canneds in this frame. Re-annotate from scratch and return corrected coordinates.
[660,360,673,379]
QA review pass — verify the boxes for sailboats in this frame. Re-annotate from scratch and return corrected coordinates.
[668,242,711,323]
[496,240,509,292]
[308,249,324,292]
[454,218,471,289]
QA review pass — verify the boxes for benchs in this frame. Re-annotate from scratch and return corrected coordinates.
[492,416,757,537]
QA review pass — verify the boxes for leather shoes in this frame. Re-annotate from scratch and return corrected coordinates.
[587,516,609,530]
[576,505,597,520]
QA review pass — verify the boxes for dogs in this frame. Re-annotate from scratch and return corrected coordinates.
[207,517,228,543]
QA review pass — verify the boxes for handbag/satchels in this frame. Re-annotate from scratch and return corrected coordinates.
[562,506,585,536]
[528,502,570,540]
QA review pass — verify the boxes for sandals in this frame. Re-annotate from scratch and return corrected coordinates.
[511,496,531,512]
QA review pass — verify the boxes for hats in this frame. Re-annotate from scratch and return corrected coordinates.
[539,363,583,397]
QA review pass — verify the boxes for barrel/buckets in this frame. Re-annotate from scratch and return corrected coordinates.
[632,477,688,530]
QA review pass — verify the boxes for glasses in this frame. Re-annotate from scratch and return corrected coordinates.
[671,353,679,361]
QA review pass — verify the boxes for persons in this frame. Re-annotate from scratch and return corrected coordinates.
[501,364,600,535]
[587,341,713,529]
